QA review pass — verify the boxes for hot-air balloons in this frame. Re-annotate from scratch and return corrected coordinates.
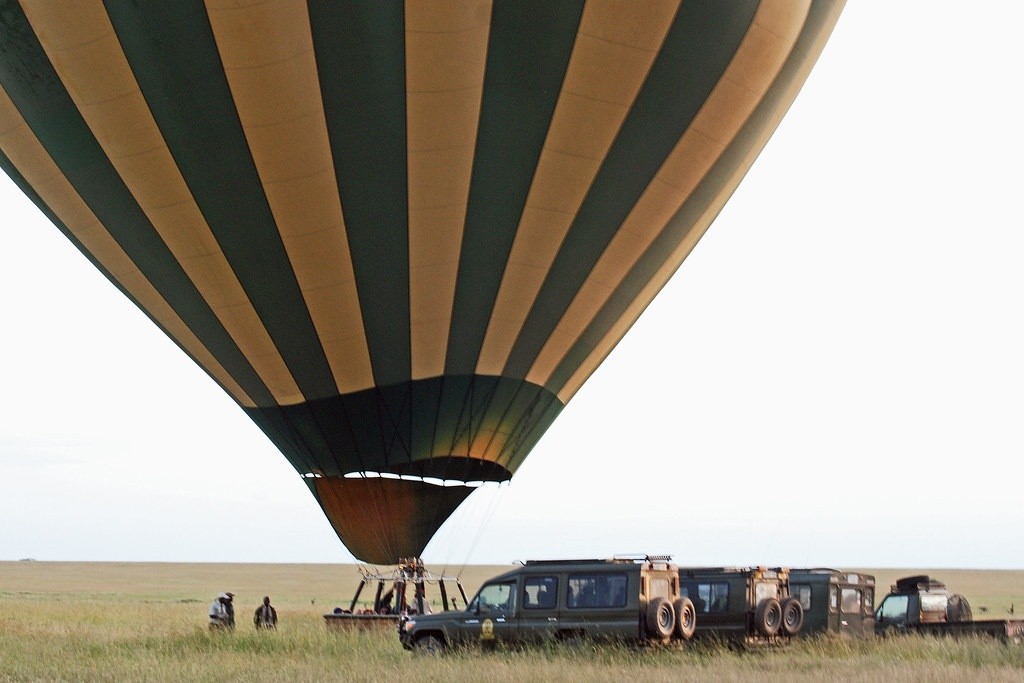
[0,0,850,640]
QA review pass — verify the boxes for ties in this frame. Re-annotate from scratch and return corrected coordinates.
[264,607,269,622]
[221,604,223,616]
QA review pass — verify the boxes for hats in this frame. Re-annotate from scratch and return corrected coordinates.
[225,592,235,597]
[218,592,230,599]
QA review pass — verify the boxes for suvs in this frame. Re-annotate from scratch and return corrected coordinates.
[792,568,877,641]
[400,555,695,656]
[679,565,804,652]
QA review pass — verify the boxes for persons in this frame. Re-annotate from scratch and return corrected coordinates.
[376,581,433,616]
[224,592,236,626]
[208,592,231,630]
[253,596,279,632]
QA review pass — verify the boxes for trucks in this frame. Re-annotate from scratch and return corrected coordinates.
[875,575,1024,647]
